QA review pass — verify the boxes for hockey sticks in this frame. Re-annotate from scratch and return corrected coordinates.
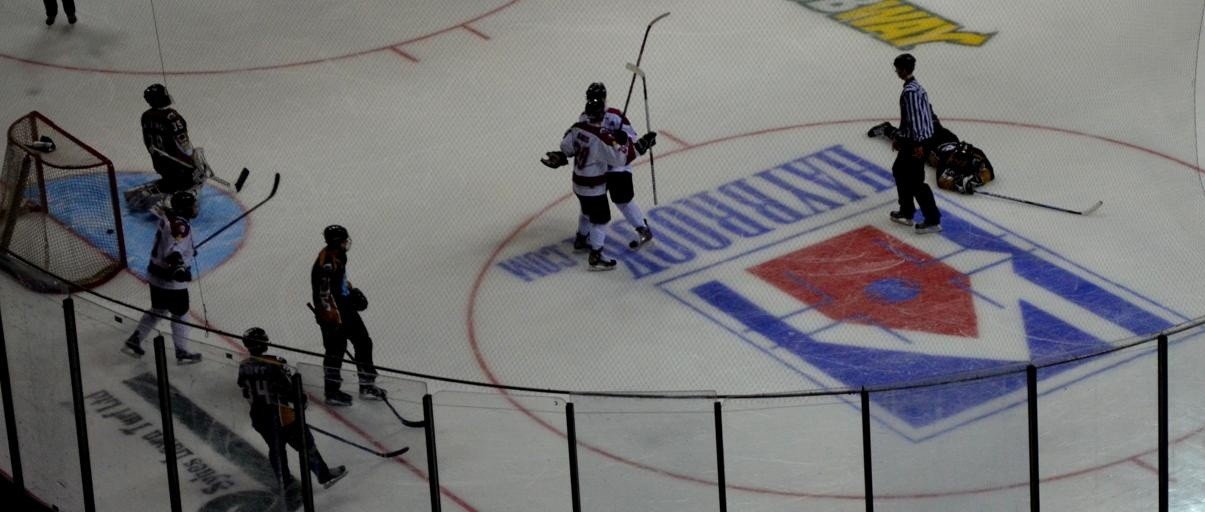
[308,423,409,457]
[618,12,671,205]
[306,303,425,428]
[974,189,1104,217]
[194,173,280,249]
[150,144,249,192]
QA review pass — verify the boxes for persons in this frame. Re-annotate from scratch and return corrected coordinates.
[890,54,941,228]
[868,104,995,193]
[43,0,77,27]
[238,329,344,480]
[125,226,201,358]
[125,84,205,236]
[311,224,388,401]
[541,83,656,249]
[562,101,630,268]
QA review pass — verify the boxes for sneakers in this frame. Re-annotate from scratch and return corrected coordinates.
[45,15,77,25]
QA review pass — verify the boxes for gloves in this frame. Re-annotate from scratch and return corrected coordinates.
[961,174,982,195]
[349,288,368,312]
[170,266,192,282]
[613,129,628,147]
[636,131,657,156]
[539,151,568,169]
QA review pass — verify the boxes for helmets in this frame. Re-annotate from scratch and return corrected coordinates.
[586,99,605,117]
[143,83,171,108]
[586,82,607,101]
[170,192,196,217]
[242,328,269,346]
[954,141,973,160]
[324,225,348,244]
[894,53,916,68]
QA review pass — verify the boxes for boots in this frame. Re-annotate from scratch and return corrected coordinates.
[124,331,145,355]
[314,462,345,485]
[573,229,618,266]
[359,376,387,397]
[628,216,655,248]
[890,206,916,220]
[175,347,201,360]
[915,212,942,229]
[868,121,894,138]
[324,379,352,402]
[279,474,303,496]
